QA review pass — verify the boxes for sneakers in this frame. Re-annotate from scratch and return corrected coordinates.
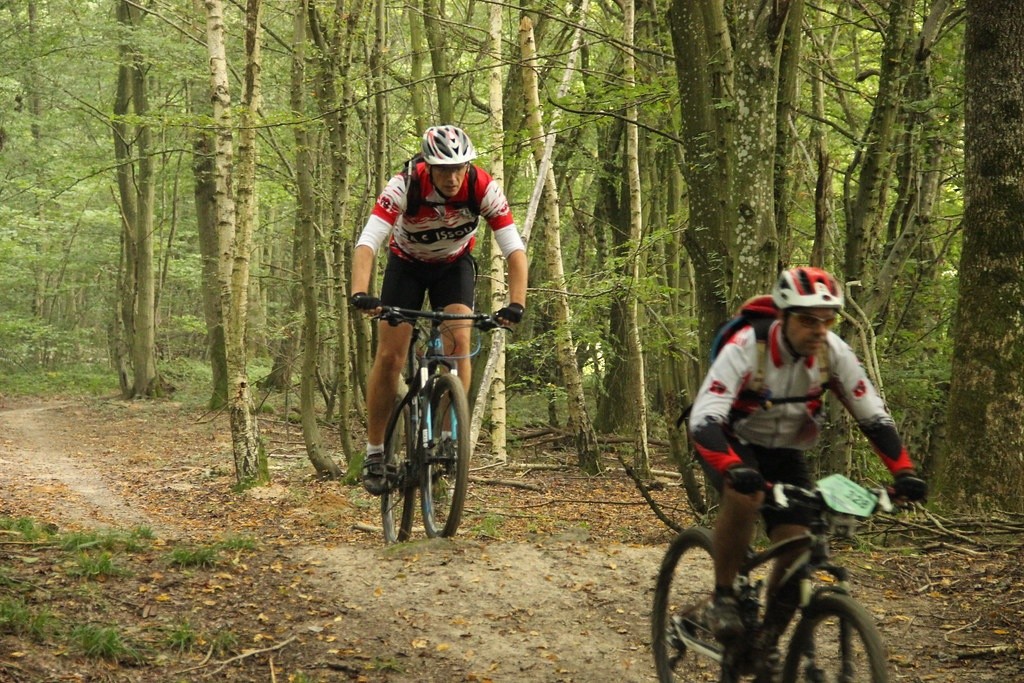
[435,438,458,472]
[362,453,386,495]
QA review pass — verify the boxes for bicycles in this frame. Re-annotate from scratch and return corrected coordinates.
[367,305,513,546]
[651,480,912,683]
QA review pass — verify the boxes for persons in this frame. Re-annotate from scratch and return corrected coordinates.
[688,266,928,683]
[350,124,528,496]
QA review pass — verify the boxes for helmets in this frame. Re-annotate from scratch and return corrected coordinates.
[771,266,845,310]
[422,125,477,165]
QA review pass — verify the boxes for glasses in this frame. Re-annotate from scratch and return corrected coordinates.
[788,311,835,329]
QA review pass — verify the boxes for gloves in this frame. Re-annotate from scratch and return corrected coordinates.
[726,467,772,495]
[888,476,928,507]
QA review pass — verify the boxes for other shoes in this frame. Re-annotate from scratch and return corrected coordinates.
[711,596,745,642]
